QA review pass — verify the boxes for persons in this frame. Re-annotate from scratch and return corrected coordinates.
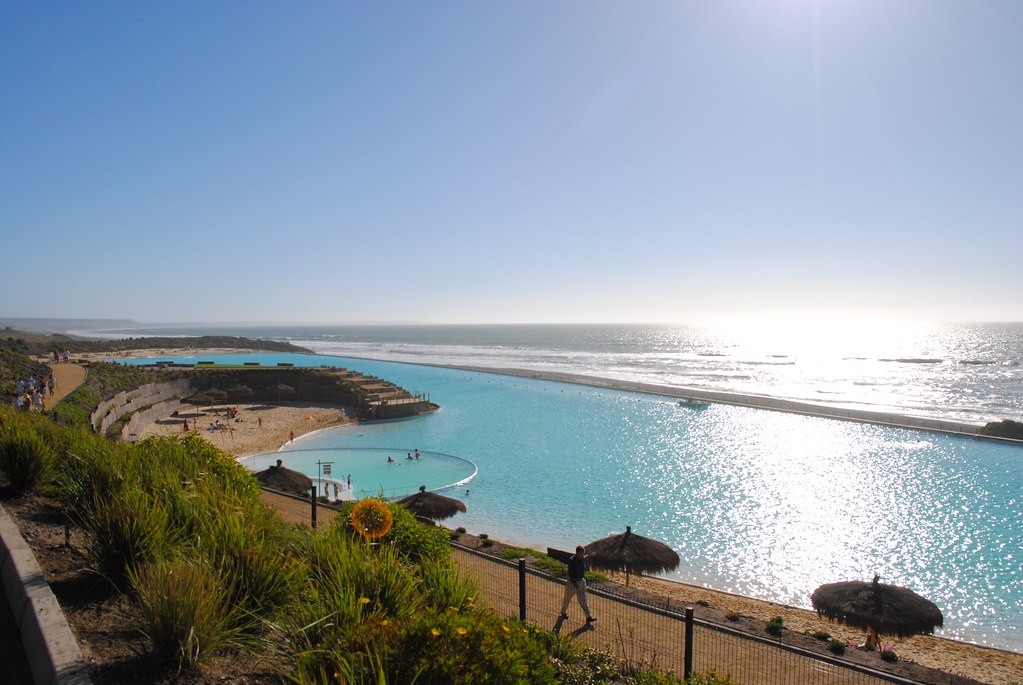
[464,489,470,496]
[54,348,70,364]
[324,473,354,499]
[14,373,56,412]
[183,407,263,432]
[387,448,421,462]
[288,430,296,443]
[846,628,882,653]
[560,545,598,623]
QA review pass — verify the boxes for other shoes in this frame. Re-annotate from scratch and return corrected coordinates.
[586,616,597,622]
[559,613,568,619]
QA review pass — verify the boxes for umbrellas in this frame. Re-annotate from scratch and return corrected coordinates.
[583,525,680,587]
[393,486,466,522]
[810,575,944,649]
[249,459,313,494]
